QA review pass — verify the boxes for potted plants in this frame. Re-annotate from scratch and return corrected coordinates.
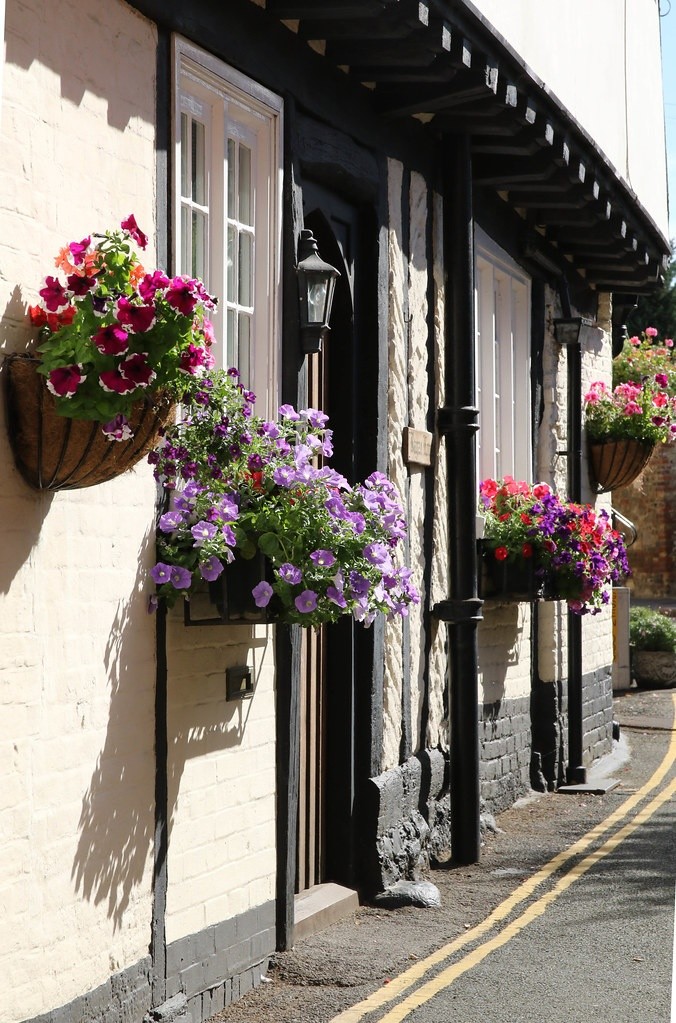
[476,537,543,603]
[628,606,676,689]
[591,436,656,494]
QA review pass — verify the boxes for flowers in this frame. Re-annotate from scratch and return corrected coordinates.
[478,476,632,616]
[26,214,218,443]
[583,373,676,443]
[147,403,420,633]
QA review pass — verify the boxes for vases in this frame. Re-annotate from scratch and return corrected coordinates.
[6,353,174,493]
[207,549,283,619]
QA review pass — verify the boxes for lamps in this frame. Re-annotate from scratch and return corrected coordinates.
[295,228,341,353]
